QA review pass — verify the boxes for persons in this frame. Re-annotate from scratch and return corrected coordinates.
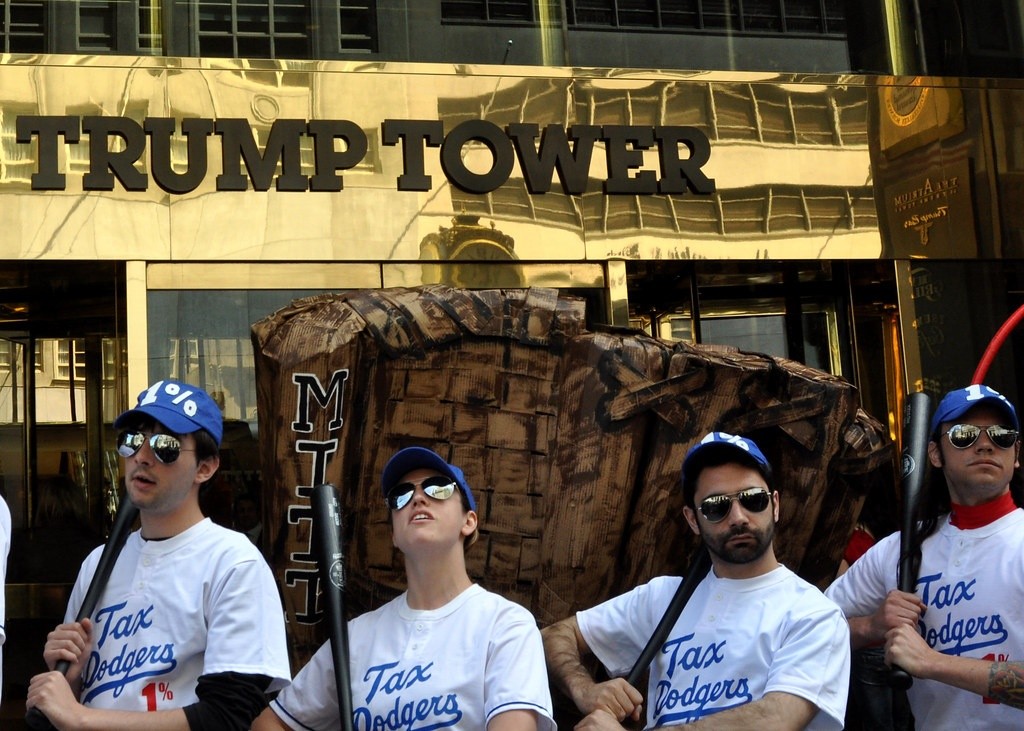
[249,448,557,731]
[825,384,1024,731]
[25,380,292,731]
[540,431,852,731]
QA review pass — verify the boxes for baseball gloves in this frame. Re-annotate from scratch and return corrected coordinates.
[246,283,889,731]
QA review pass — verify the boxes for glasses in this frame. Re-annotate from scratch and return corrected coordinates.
[116,430,197,464]
[940,423,1019,449]
[694,487,771,523]
[383,475,463,513]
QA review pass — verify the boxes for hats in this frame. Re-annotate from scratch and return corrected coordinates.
[382,447,475,512]
[113,381,223,448]
[929,384,1018,442]
[682,432,769,481]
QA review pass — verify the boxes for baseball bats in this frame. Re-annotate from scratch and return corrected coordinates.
[624,538,711,686]
[888,392,934,691]
[310,484,354,731]
[24,495,139,728]
